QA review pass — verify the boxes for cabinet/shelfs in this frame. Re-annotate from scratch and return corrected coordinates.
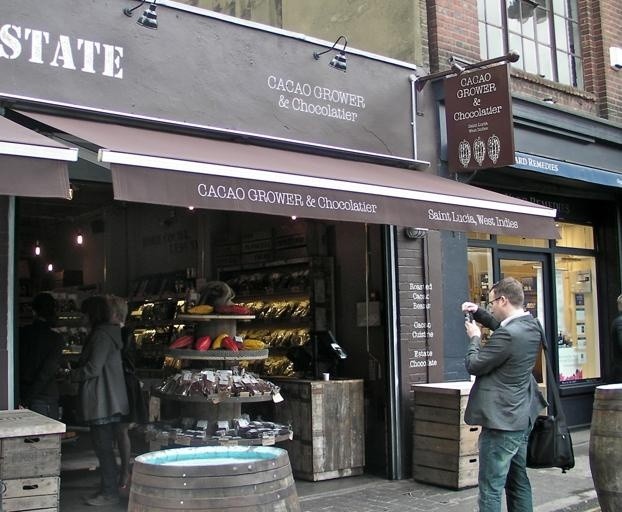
[128,257,317,451]
[0,408,68,512]
[16,270,95,433]
[270,378,367,484]
[405,379,487,490]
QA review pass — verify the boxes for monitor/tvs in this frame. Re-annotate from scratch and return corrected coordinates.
[308,330,346,364]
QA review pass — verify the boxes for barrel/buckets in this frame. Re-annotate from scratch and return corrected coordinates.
[127,447,301,512]
[588,384,622,508]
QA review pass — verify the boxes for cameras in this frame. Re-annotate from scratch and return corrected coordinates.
[464,311,474,323]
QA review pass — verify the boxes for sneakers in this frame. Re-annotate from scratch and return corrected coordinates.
[78,481,129,508]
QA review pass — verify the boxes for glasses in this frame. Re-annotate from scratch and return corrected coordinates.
[487,297,502,307]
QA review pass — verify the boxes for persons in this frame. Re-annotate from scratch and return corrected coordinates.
[610,293,621,386]
[105,294,134,494]
[16,291,66,424]
[56,295,126,508]
[461,277,550,512]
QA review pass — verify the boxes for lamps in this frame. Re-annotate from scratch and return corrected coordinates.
[122,0,160,31]
[406,227,430,239]
[312,35,350,73]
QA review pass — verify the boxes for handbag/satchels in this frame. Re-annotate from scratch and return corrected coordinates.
[527,414,575,474]
